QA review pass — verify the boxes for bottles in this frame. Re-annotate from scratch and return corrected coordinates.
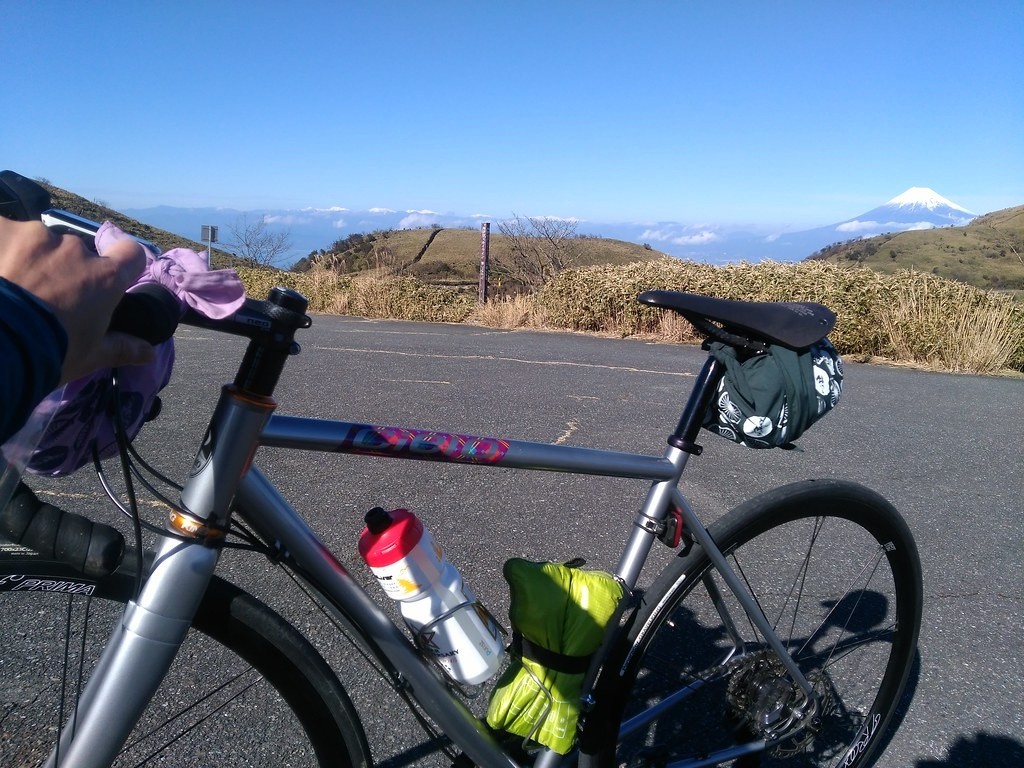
[358,507,504,685]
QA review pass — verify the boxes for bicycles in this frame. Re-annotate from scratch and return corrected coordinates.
[0,170,923,760]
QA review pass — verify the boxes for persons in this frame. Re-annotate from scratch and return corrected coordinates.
[0,216,155,448]
